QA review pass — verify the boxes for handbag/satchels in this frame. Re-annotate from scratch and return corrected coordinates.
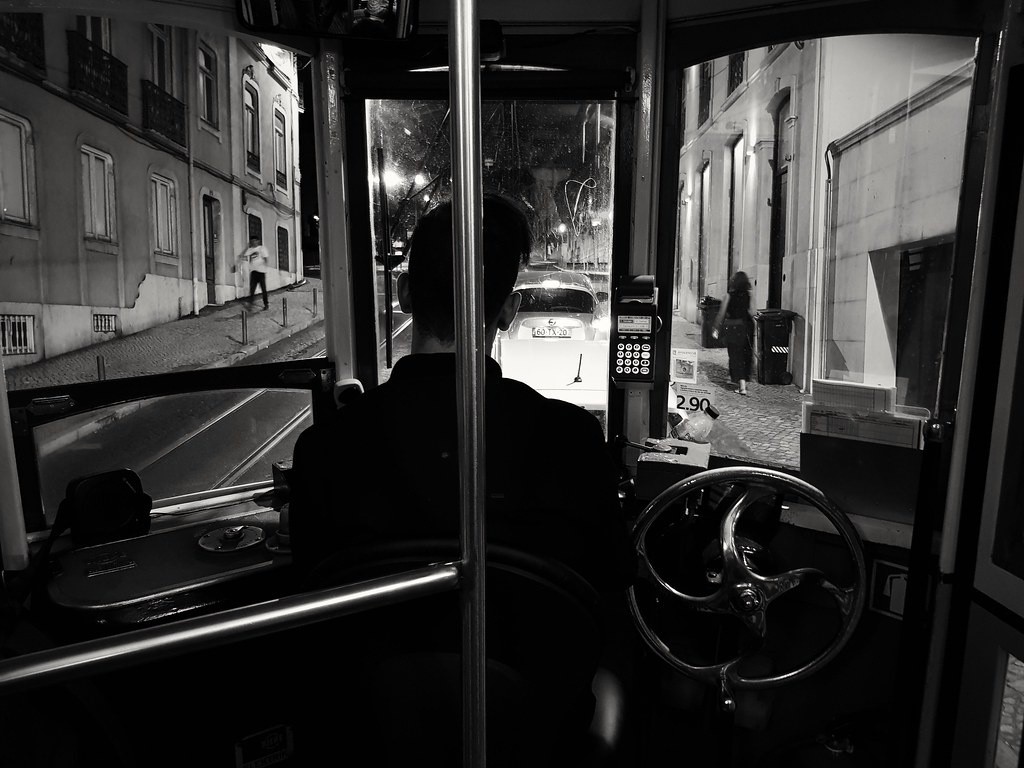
[722,318,746,346]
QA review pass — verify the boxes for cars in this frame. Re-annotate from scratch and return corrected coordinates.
[495,269,609,343]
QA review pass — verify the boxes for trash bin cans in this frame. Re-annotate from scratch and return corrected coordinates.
[697,302,722,348]
[753,308,798,385]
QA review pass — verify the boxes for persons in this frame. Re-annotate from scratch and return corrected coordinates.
[293,192,631,768]
[713,271,756,397]
[241,237,273,311]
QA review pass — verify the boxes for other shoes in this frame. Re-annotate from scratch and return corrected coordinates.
[734,389,747,395]
[242,302,252,312]
[262,305,269,310]
